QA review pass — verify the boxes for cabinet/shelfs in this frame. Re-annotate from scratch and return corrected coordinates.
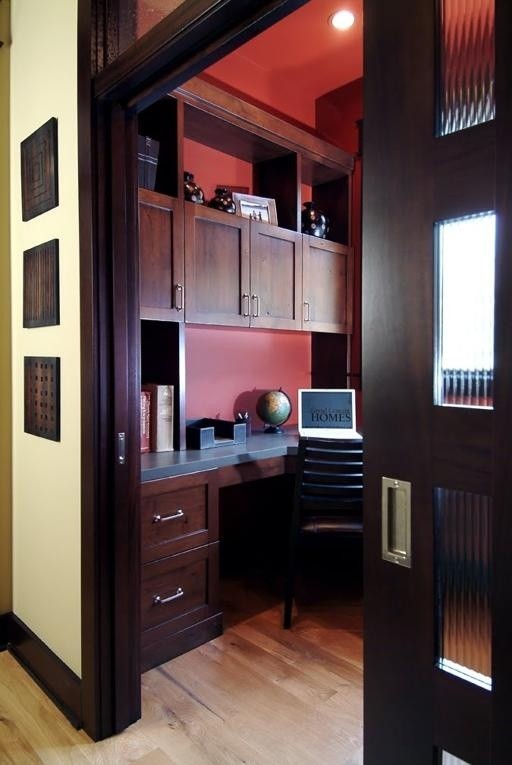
[139,75,354,246]
[141,309,353,453]
[303,233,351,336]
[184,199,302,331]
[138,186,185,322]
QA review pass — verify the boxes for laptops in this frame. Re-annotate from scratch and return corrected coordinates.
[297,389,363,440]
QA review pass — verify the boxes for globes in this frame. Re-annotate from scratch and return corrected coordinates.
[257,387,292,434]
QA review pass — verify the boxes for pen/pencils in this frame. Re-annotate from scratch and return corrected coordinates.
[238,411,248,418]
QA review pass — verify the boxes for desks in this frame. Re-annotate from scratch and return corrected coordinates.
[140,426,364,672]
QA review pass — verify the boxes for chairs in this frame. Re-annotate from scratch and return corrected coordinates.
[284,438,365,628]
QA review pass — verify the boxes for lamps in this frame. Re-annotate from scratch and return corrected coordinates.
[331,9,355,30]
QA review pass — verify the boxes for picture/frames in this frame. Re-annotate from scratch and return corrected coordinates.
[232,192,278,228]
